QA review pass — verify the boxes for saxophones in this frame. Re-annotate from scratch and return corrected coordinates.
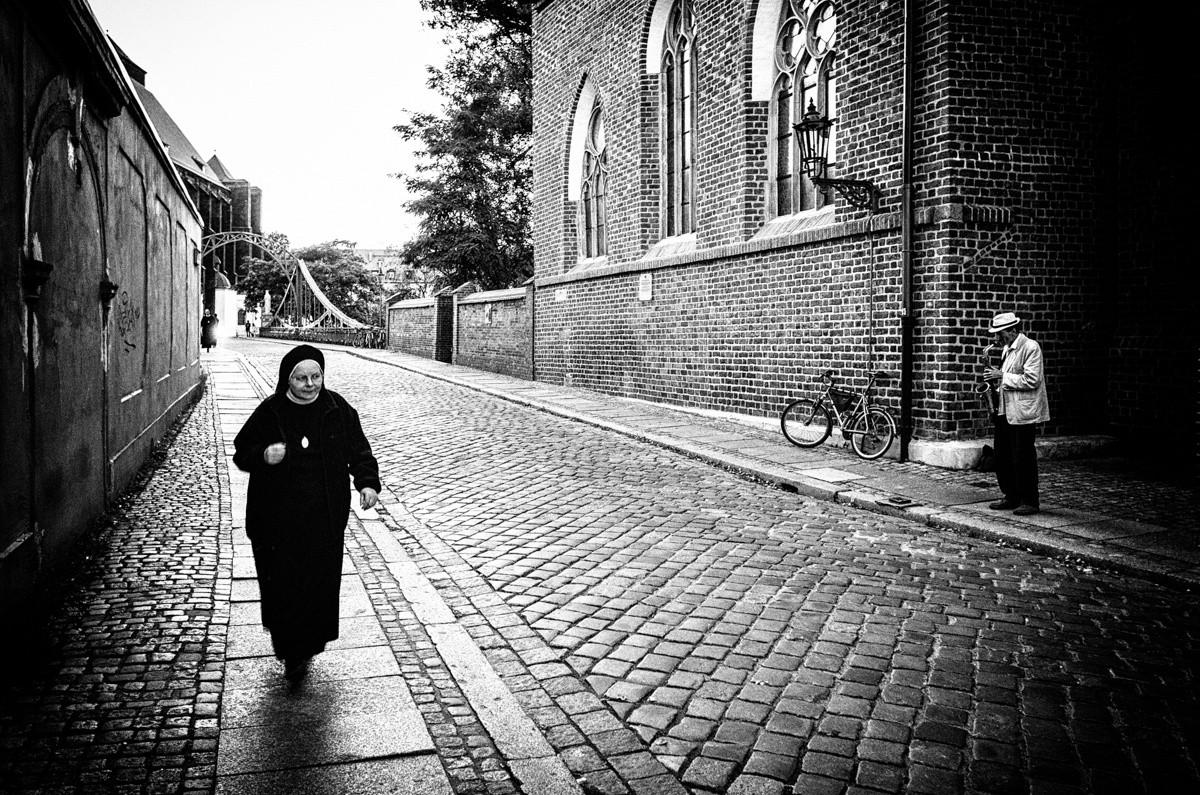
[975,339,1000,415]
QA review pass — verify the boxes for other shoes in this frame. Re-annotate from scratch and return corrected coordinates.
[1013,504,1040,516]
[288,683,305,700]
[989,498,1021,510]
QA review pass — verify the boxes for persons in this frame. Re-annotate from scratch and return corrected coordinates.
[233,345,381,684]
[989,311,1050,516]
[245,320,251,337]
[200,307,218,352]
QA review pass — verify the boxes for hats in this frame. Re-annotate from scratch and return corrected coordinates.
[988,312,1021,334]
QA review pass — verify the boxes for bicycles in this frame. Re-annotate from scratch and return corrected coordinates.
[353,327,385,349]
[780,368,899,460]
